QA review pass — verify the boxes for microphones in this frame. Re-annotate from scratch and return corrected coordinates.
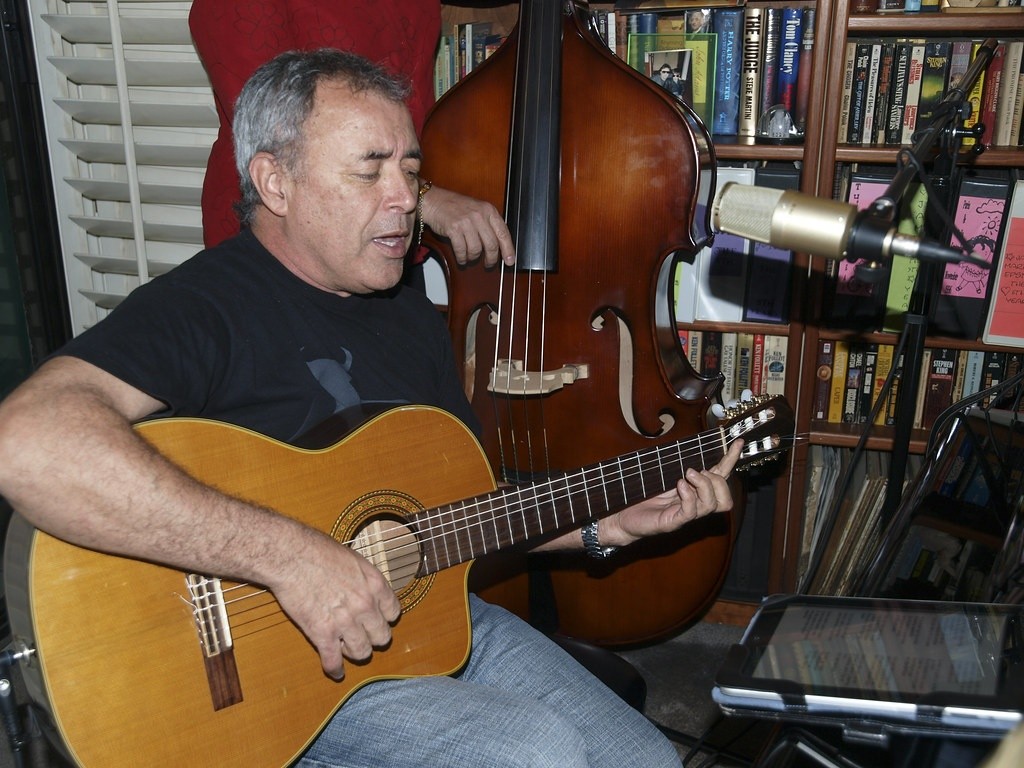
[712,182,965,265]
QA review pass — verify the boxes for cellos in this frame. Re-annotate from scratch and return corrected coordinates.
[407,1,747,653]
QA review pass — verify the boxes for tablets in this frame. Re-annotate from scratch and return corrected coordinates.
[712,594,1024,733]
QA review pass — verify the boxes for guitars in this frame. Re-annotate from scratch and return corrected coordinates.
[2,387,798,767]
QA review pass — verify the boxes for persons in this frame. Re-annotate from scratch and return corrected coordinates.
[651,60,671,86]
[0,46,744,768]
[664,67,685,100]
[688,11,710,41]
[189,0,519,269]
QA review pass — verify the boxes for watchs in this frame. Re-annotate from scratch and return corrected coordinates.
[580,521,620,558]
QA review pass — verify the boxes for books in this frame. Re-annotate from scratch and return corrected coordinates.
[434,0,1024,149]
[677,330,1024,598]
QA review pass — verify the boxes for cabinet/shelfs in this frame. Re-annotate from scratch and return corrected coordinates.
[444,2,1023,615]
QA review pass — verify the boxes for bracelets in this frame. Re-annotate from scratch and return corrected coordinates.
[416,180,431,245]
